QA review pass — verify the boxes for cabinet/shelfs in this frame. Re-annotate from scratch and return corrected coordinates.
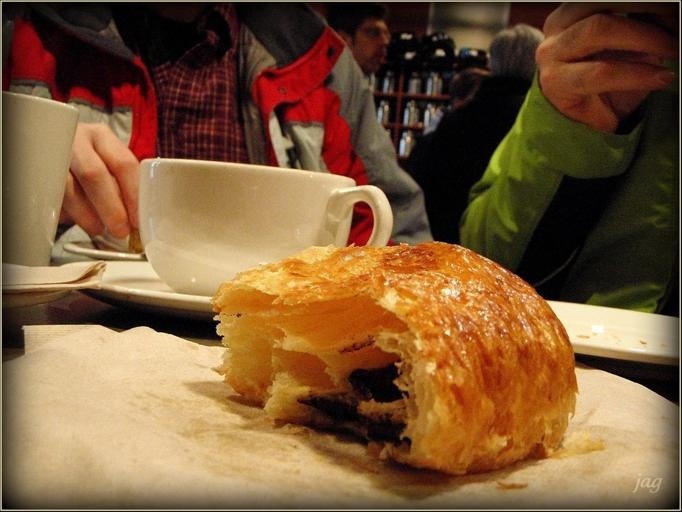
[364,63,452,167]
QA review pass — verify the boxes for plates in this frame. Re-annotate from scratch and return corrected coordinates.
[2,289,71,314]
[61,236,144,260]
[59,259,217,320]
[544,299,679,363]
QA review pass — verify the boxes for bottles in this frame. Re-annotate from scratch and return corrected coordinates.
[375,67,446,160]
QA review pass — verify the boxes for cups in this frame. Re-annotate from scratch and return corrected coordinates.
[138,156,396,295]
[2,91,80,267]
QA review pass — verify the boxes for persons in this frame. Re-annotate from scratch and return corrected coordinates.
[2,3,436,264]
[326,2,390,76]
[456,3,679,319]
[400,20,543,243]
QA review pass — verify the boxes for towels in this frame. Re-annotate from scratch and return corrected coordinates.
[3,259,108,291]
[3,323,679,511]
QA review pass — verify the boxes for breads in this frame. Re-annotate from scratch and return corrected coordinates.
[214,241,578,476]
